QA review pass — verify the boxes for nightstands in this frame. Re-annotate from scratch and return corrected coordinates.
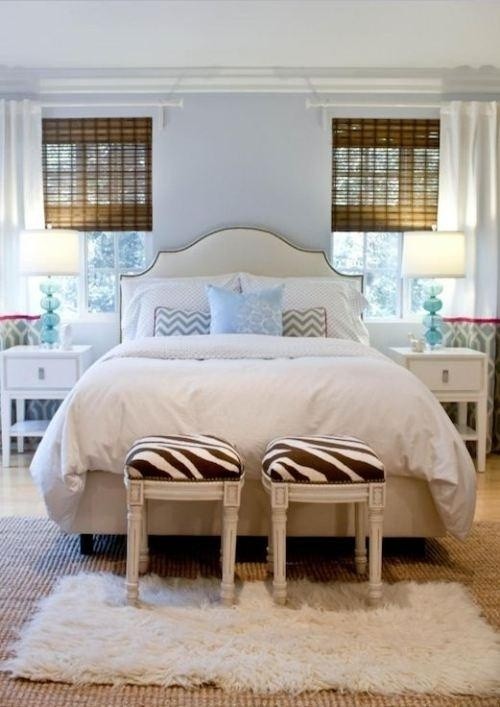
[0,343,94,468]
[388,346,490,472]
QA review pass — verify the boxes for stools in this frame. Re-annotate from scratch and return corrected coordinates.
[121,433,245,608]
[259,432,388,608]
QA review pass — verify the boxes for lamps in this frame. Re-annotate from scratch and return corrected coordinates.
[399,229,468,352]
[11,226,82,347]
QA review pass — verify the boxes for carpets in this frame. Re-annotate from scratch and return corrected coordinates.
[0,568,500,696]
[0,513,500,706]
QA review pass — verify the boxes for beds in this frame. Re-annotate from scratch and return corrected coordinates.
[61,226,451,559]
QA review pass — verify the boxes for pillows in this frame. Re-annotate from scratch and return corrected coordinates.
[135,271,352,340]
